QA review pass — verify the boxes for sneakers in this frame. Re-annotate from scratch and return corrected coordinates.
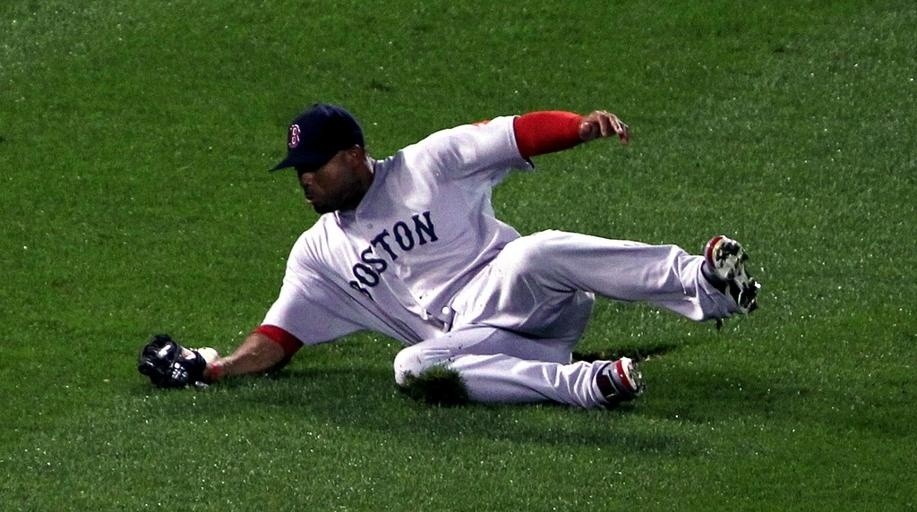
[701,235,758,314]
[602,356,646,401]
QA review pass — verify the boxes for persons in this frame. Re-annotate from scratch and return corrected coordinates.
[138,102,759,411]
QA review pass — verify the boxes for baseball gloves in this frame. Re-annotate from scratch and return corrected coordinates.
[138,334,205,389]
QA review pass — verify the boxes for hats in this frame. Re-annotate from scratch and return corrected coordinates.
[268,103,363,173]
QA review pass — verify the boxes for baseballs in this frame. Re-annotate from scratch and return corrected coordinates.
[198,347,220,364]
